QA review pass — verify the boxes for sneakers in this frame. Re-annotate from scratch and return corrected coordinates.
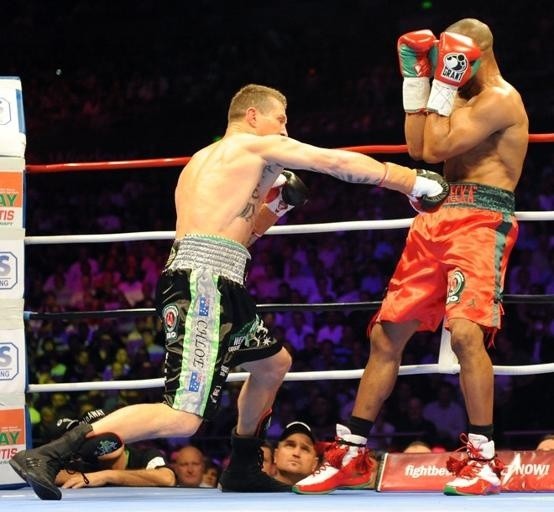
[442,431,503,497]
[290,421,375,496]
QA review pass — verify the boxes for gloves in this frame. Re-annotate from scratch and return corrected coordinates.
[261,167,311,223]
[406,168,450,215]
[394,28,438,80]
[433,29,482,87]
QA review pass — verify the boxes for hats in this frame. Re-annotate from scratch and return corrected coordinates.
[278,420,316,443]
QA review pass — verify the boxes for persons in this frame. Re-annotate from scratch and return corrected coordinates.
[289,18,530,494]
[23,229,554,489]
[9,85,449,500]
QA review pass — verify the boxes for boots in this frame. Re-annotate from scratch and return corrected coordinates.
[218,429,292,494]
[9,420,123,501]
